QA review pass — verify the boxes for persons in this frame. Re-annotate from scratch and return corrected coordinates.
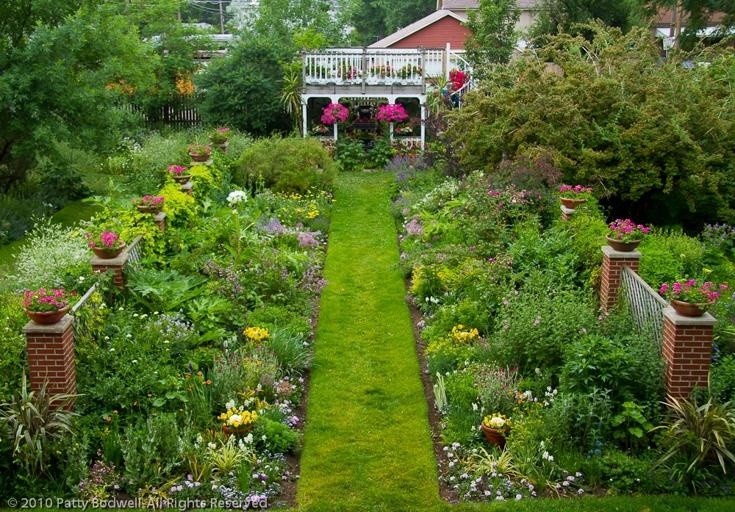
[448,66,466,106]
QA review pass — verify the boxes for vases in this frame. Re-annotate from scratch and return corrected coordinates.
[605,233,642,252]
[559,197,588,210]
[481,423,511,448]
[667,296,717,316]
[223,425,253,438]
[24,307,71,325]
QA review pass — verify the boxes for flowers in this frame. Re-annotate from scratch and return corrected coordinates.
[607,218,650,241]
[219,402,257,426]
[659,276,726,303]
[243,326,270,344]
[321,102,350,126]
[448,323,478,344]
[559,184,591,200]
[377,104,409,124]
[20,125,247,313]
[482,412,511,429]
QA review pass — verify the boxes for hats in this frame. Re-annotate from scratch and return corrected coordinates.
[453,67,459,73]
